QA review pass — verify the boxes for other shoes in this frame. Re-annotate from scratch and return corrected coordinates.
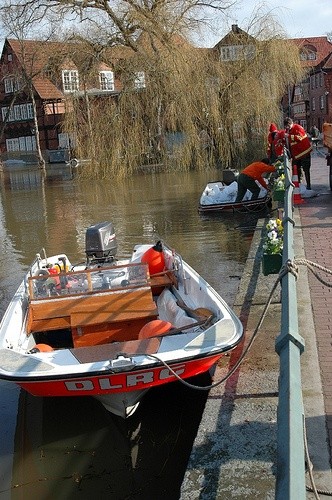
[306,184,311,191]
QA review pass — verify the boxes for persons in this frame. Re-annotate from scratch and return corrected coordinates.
[235,158,278,203]
[284,117,312,188]
[310,124,320,151]
[267,122,285,165]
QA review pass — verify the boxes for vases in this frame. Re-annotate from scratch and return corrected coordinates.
[261,254,282,277]
[272,190,285,201]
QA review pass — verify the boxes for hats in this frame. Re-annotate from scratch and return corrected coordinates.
[269,124,278,133]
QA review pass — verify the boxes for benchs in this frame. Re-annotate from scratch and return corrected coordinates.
[25,287,159,335]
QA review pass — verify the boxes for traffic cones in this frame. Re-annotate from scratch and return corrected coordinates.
[292,164,307,205]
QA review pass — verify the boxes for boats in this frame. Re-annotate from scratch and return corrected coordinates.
[198,168,272,212]
[0,221,245,398]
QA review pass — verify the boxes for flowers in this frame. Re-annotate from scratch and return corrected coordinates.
[261,218,284,255]
[274,173,286,191]
